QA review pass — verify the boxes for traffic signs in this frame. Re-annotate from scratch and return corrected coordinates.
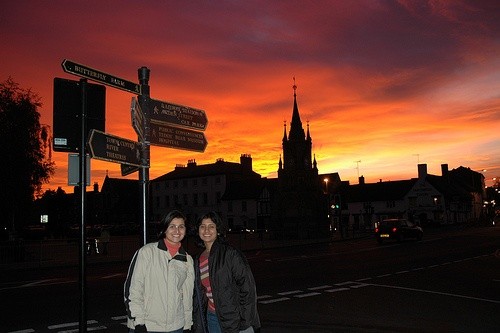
[61,58,142,95]
[131,97,146,141]
[86,129,150,168]
[148,97,208,131]
[149,122,208,153]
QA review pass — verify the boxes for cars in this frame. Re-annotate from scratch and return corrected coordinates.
[0,219,131,259]
[373,218,424,245]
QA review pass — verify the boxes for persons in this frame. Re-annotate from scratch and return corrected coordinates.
[123,210,195,333]
[192,211,261,333]
